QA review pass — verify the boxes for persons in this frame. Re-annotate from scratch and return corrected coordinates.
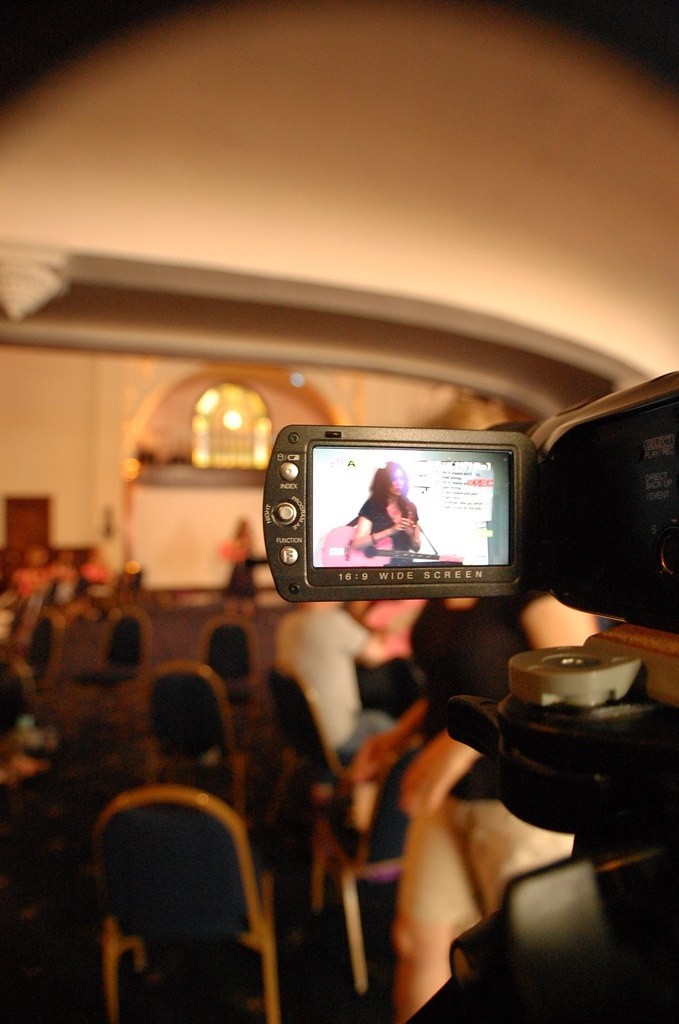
[352,461,421,566]
[347,594,601,1024]
[272,601,412,765]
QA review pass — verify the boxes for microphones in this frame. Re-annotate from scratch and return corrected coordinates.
[409,510,438,554]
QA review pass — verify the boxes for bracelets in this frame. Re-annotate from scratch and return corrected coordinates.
[372,533,376,545]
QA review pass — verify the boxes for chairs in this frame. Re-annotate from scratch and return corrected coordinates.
[0,560,406,1024]
